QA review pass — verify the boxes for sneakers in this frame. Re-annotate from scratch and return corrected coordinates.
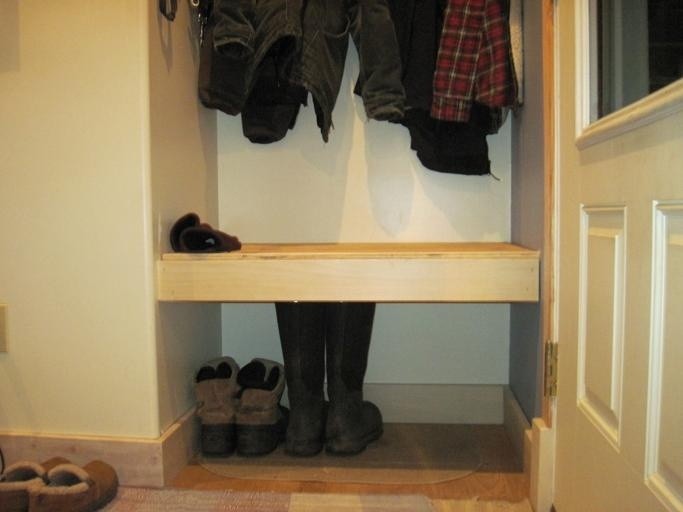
[1,457,71,512]
[26,461,117,511]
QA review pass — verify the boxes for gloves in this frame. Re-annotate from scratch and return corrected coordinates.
[170,213,242,253]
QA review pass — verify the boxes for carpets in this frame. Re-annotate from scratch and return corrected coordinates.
[196,427,484,482]
[97,487,531,512]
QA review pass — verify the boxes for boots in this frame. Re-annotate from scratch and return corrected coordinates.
[274,302,328,457]
[236,357,288,456]
[324,302,382,456]
[193,356,240,459]
